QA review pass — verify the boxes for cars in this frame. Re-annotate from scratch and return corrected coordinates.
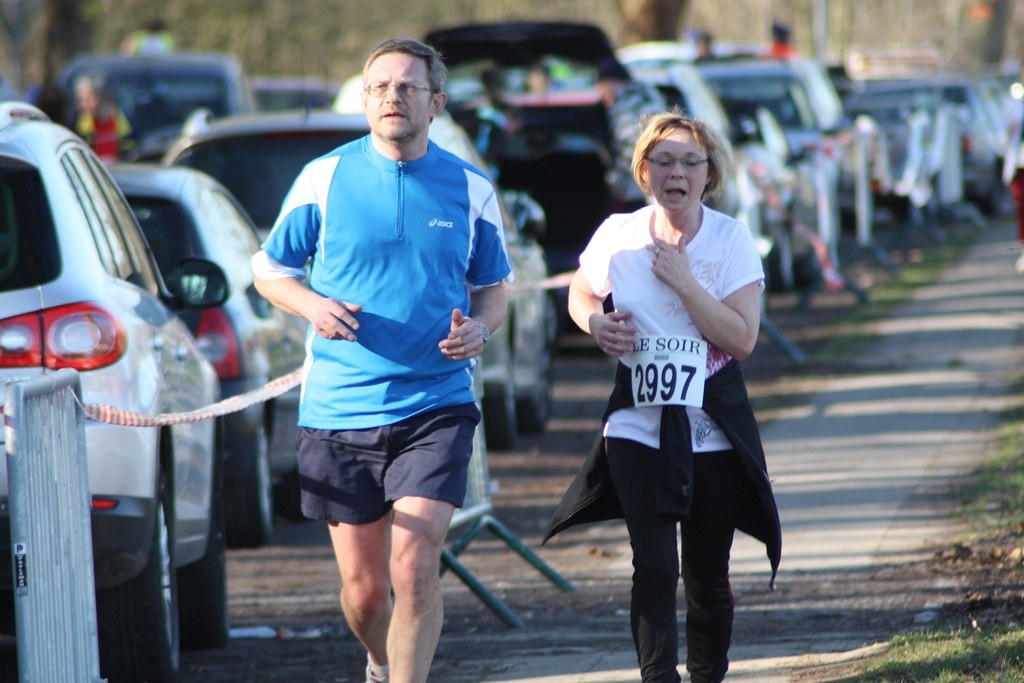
[252,17,1024,387]
[104,163,296,553]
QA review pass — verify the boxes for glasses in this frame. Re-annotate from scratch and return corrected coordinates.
[645,152,708,170]
[365,80,433,99]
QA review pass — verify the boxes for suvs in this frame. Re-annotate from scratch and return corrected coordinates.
[153,107,573,502]
[39,52,249,169]
[0,100,237,682]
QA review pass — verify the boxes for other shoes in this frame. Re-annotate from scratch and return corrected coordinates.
[364,664,389,683]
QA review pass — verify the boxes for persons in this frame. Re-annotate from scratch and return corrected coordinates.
[767,22,796,56]
[692,34,717,61]
[598,61,670,206]
[76,78,133,162]
[461,60,595,175]
[1002,63,1024,272]
[541,112,781,683]
[118,19,177,56]
[251,38,516,682]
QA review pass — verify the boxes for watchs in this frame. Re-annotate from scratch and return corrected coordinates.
[478,321,490,342]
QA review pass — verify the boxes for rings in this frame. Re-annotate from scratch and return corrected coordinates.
[656,247,660,254]
[653,258,657,262]
